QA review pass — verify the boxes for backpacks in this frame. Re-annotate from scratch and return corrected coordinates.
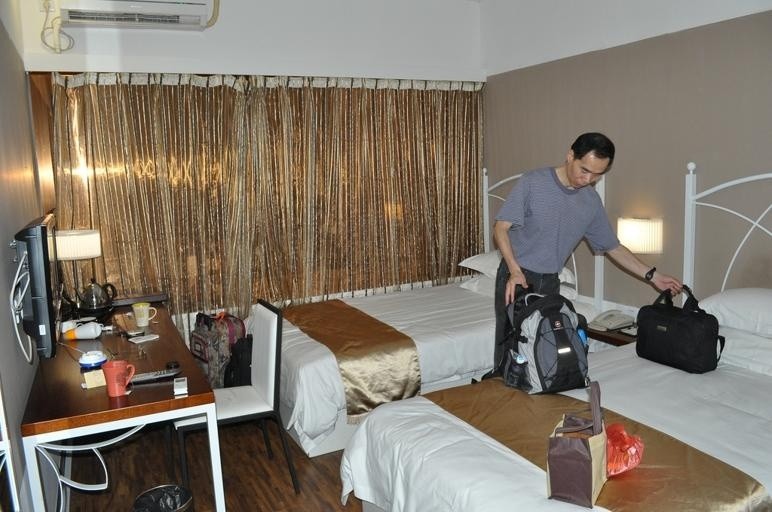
[223,335,253,385]
[501,286,592,396]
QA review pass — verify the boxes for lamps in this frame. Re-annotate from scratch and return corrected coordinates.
[55,230,101,307]
[616,217,663,254]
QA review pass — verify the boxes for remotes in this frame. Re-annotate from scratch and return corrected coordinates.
[172,377,188,397]
[132,368,182,385]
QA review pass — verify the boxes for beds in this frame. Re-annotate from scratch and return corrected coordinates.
[340,161,772,512]
[242,167,605,459]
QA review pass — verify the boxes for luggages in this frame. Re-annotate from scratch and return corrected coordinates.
[190,312,245,385]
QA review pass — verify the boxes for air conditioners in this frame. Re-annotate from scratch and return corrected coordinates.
[55,0,221,31]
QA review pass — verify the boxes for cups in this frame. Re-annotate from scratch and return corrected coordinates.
[131,303,157,327]
[102,360,136,396]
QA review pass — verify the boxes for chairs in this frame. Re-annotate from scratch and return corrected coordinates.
[165,298,301,495]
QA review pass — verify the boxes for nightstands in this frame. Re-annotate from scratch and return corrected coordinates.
[588,324,637,346]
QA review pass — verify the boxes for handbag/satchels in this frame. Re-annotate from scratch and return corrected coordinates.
[547,411,608,509]
[635,304,719,376]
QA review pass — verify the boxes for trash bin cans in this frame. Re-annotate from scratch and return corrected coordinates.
[133,484,194,512]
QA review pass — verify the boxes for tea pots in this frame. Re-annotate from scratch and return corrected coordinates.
[72,279,118,315]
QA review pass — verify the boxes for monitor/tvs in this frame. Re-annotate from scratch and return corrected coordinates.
[16,213,62,359]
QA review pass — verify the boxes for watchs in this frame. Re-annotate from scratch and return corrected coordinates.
[642,267,657,282]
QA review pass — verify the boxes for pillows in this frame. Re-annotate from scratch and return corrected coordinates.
[698,287,772,376]
[457,249,579,300]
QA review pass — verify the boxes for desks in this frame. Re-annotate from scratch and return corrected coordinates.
[20,304,228,512]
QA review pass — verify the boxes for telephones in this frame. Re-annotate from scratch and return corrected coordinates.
[587,309,634,332]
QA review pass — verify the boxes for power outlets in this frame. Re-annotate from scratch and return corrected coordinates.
[40,1,54,12]
[14,293,24,324]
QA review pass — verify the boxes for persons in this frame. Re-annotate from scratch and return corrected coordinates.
[494,132,683,376]
